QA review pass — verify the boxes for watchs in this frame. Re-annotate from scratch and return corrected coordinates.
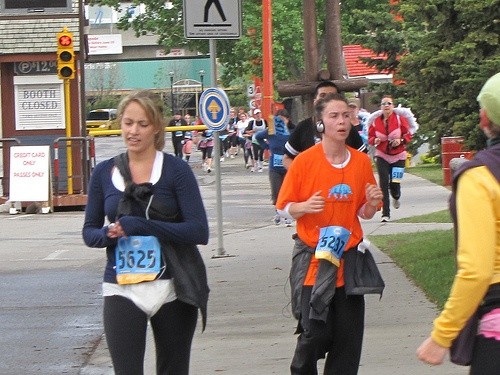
[400,137,404,145]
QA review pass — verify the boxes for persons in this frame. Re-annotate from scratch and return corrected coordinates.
[366,93,413,222]
[274,92,386,375]
[282,79,370,175]
[415,70,500,375]
[245,109,269,173]
[191,106,256,174]
[343,101,369,157]
[80,91,211,375]
[255,109,302,227]
[168,112,187,161]
[180,114,197,164]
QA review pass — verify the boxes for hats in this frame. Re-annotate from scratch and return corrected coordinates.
[253,107,262,116]
[346,98,361,110]
[476,69,499,129]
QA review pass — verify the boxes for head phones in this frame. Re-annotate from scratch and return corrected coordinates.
[316,119,324,133]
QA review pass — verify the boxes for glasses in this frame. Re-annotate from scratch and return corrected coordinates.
[380,101,394,107]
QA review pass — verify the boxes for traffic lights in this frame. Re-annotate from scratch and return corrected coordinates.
[56,32,75,80]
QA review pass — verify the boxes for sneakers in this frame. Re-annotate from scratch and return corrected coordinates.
[234,148,241,156]
[201,163,212,175]
[381,215,389,221]
[284,218,293,228]
[273,209,281,225]
[249,167,264,173]
[225,151,229,157]
[392,197,400,210]
[220,156,224,162]
[244,163,250,169]
[230,156,236,161]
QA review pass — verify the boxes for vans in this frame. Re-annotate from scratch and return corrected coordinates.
[86,108,121,137]
